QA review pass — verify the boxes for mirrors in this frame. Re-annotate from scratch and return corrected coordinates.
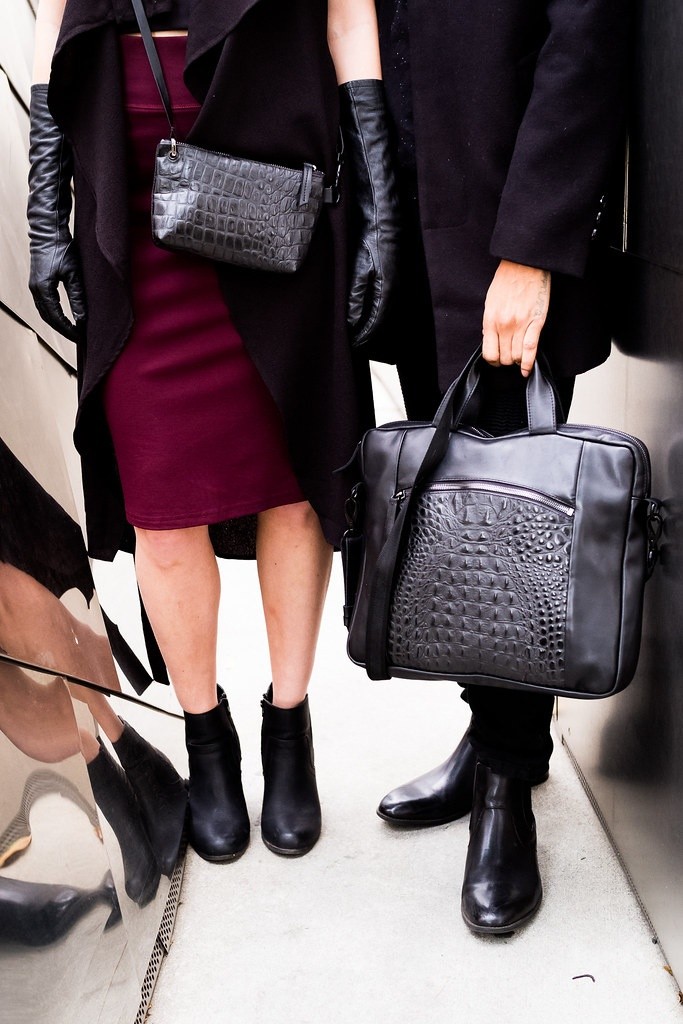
[0,0,194,1024]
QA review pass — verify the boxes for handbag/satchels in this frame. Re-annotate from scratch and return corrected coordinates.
[150,139,325,273]
[340,341,661,701]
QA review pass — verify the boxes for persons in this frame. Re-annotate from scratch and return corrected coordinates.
[368,0,683,936]
[0,438,189,947]
[26,0,412,864]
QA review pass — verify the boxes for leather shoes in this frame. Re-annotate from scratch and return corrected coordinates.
[461,761,543,933]
[377,714,554,824]
[0,876,87,944]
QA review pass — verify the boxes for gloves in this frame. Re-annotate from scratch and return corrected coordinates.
[28,85,86,346]
[338,82,414,346]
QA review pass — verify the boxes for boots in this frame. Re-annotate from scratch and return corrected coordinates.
[260,683,322,855]
[110,713,185,877]
[185,683,251,861]
[86,736,160,907]
